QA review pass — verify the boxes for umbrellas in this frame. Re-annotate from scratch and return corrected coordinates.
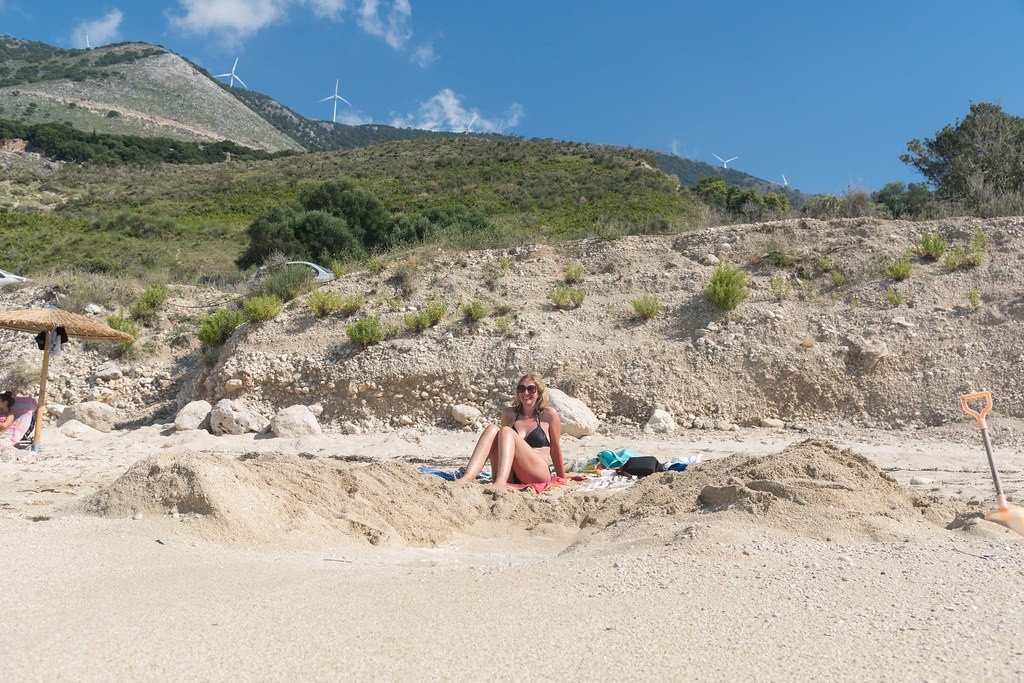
[0,310,133,452]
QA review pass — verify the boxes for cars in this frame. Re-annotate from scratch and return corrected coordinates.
[0,269,29,286]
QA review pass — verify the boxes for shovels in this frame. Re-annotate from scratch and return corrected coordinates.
[960,392,1024,537]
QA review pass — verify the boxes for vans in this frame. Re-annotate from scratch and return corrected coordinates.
[253,260,335,286]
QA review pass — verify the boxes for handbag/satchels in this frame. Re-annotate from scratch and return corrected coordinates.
[619,456,663,476]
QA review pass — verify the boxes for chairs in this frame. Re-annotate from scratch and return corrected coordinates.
[0,397,38,447]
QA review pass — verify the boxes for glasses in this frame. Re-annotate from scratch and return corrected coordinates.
[517,385,538,394]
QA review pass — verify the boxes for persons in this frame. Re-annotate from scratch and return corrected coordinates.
[0,391,15,435]
[452,374,576,494]
